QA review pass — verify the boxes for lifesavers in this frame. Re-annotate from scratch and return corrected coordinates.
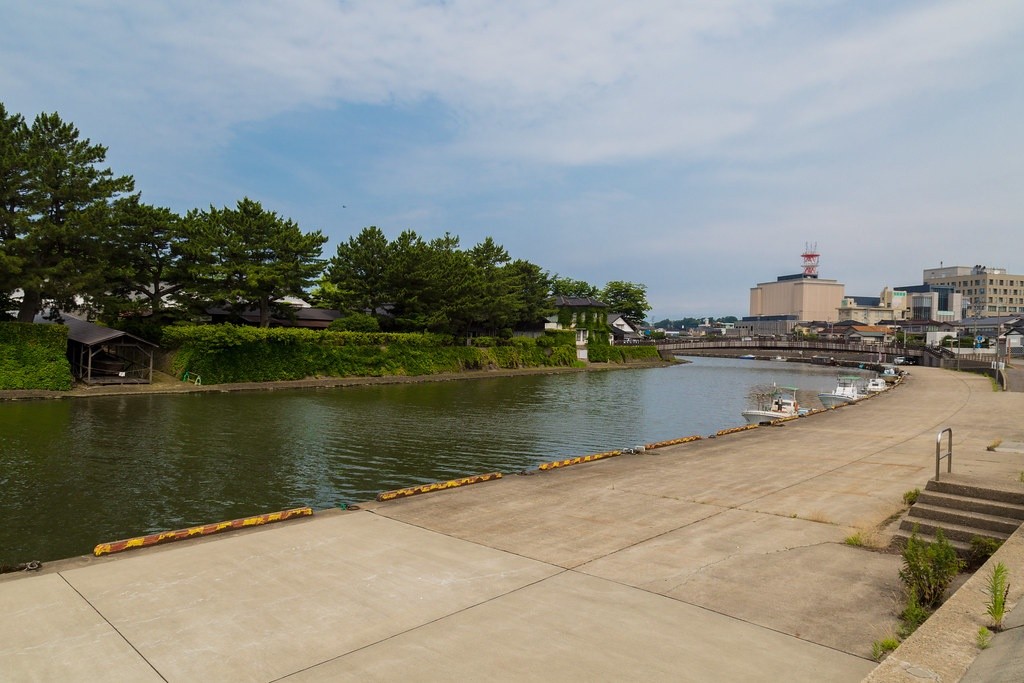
[794,402,798,411]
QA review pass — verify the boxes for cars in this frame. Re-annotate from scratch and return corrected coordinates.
[893,356,906,365]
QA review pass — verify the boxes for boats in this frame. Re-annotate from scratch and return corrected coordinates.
[739,354,756,360]
[740,381,818,425]
[818,374,868,410]
[859,378,891,395]
[879,368,899,383]
[769,356,787,362]
